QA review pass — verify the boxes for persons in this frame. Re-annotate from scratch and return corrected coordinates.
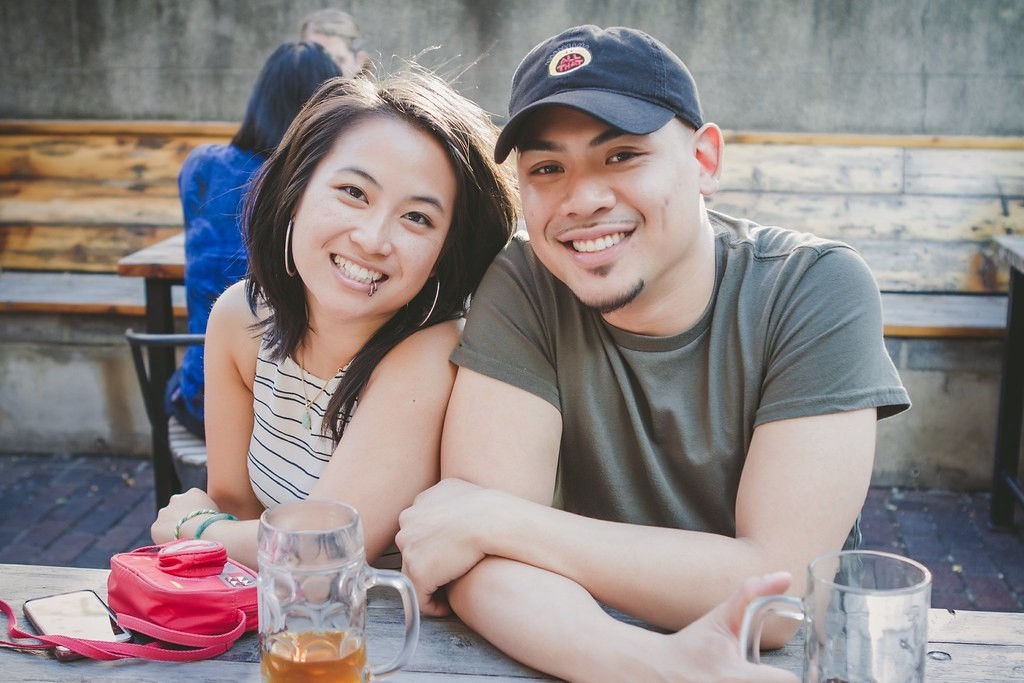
[151,37,519,617]
[298,7,368,80]
[395,24,912,683]
[164,41,344,442]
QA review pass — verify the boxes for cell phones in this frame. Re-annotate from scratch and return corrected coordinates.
[23,589,134,662]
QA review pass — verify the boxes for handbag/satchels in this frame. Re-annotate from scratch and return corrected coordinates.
[106,538,260,636]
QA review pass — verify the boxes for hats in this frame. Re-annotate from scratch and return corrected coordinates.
[493,23,704,163]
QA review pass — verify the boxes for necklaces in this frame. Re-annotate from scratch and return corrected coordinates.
[300,344,338,430]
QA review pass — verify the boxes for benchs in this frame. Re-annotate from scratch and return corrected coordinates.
[0,116,1024,341]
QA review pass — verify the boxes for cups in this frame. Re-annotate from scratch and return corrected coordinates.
[258,498,420,683]
[740,550,932,683]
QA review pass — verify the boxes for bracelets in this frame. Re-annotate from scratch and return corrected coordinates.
[194,513,239,540]
[174,508,219,540]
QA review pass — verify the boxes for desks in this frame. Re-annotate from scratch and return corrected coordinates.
[116,232,185,494]
[988,232,1024,535]
[0,564,1024,683]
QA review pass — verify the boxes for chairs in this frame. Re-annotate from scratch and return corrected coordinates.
[126,328,205,519]
